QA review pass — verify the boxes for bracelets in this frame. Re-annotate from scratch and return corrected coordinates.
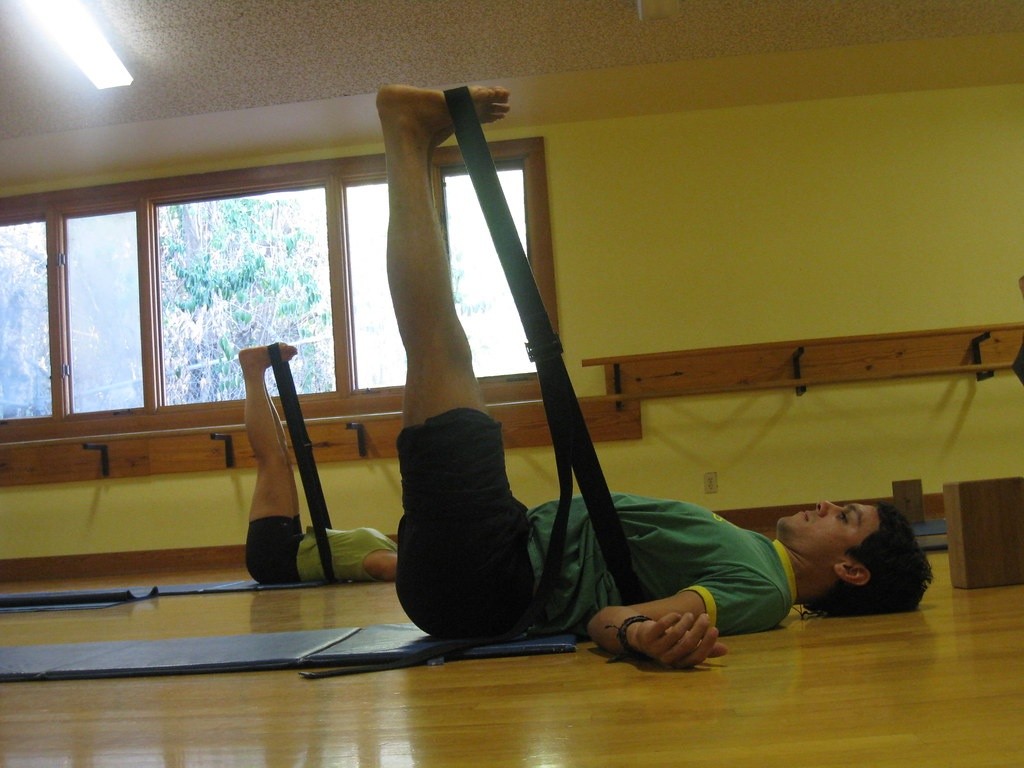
[602,615,652,664]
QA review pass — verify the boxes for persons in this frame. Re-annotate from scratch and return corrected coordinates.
[238,343,398,585]
[376,85,933,668]
